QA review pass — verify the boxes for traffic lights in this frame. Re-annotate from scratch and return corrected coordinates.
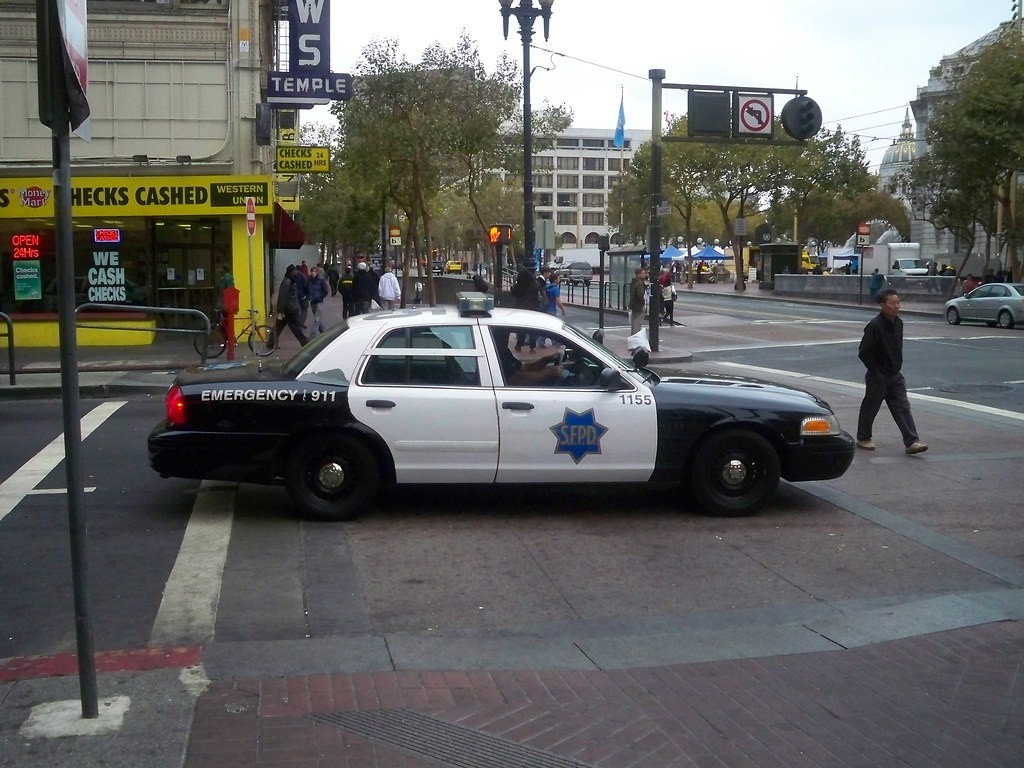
[490,224,513,244]
[781,97,822,139]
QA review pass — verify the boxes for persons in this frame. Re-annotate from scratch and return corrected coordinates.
[926,263,956,293]
[215,264,239,348]
[510,266,566,353]
[473,275,488,293]
[641,259,724,284]
[819,260,822,265]
[266,259,402,349]
[960,273,974,296]
[492,327,564,387]
[869,268,885,304]
[841,262,858,274]
[659,268,677,327]
[856,290,929,455]
[977,266,1013,287]
[628,268,647,335]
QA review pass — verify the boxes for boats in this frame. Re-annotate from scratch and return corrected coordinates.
[144,290,857,518]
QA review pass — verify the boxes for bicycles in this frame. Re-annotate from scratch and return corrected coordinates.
[193,309,278,359]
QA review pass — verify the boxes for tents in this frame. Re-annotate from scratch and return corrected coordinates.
[684,245,734,283]
[817,251,828,260]
[640,245,730,261]
[833,248,858,274]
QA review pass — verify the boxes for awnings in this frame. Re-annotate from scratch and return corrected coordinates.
[271,201,306,250]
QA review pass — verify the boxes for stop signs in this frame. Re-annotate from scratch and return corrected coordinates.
[246,196,256,236]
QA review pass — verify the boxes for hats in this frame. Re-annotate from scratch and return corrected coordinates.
[357,263,366,269]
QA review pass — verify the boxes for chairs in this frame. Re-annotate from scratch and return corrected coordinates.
[443,341,475,386]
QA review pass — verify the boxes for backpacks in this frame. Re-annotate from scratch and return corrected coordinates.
[539,285,557,312]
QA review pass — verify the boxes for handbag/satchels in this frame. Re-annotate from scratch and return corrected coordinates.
[671,284,678,301]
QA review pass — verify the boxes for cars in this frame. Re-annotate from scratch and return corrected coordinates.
[424,261,443,275]
[444,260,461,275]
[554,261,593,286]
[943,283,1024,329]
[45,275,153,312]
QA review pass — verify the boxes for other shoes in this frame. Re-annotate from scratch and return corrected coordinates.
[855,439,875,449]
[530,349,536,353]
[905,442,928,454]
[514,346,521,351]
[267,343,280,349]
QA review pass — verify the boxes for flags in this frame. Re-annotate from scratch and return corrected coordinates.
[613,88,626,149]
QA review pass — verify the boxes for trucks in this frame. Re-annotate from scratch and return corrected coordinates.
[858,242,930,276]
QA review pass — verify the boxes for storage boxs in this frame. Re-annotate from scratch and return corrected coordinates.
[748,268,756,281]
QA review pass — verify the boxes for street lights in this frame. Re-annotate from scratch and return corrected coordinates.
[500,0,556,315]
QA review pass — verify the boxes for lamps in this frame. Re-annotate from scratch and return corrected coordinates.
[176,155,192,165]
[133,154,148,165]
